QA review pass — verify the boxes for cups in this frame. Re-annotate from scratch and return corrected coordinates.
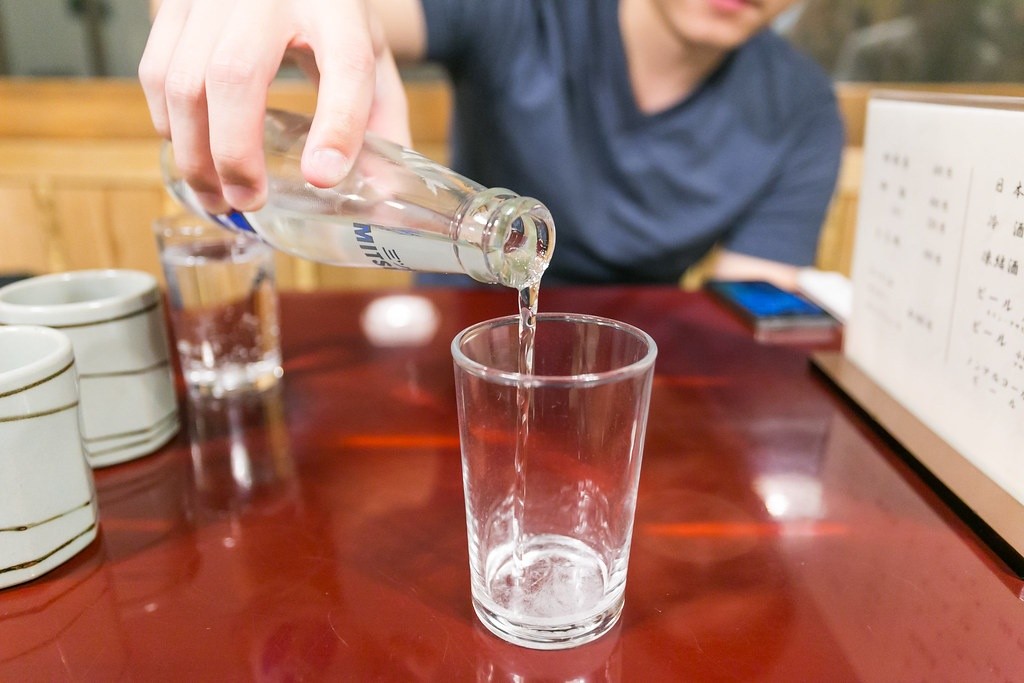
[451,312,658,651]
[153,213,284,411]
[0,266,183,469]
[0,324,100,590]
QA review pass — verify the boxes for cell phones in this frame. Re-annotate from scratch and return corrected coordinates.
[701,278,836,336]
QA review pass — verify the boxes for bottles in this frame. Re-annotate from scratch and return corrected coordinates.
[159,105,557,289]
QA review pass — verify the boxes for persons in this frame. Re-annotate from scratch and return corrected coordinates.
[138,1,844,294]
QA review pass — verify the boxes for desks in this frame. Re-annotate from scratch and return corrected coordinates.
[0,276,1024,683]
